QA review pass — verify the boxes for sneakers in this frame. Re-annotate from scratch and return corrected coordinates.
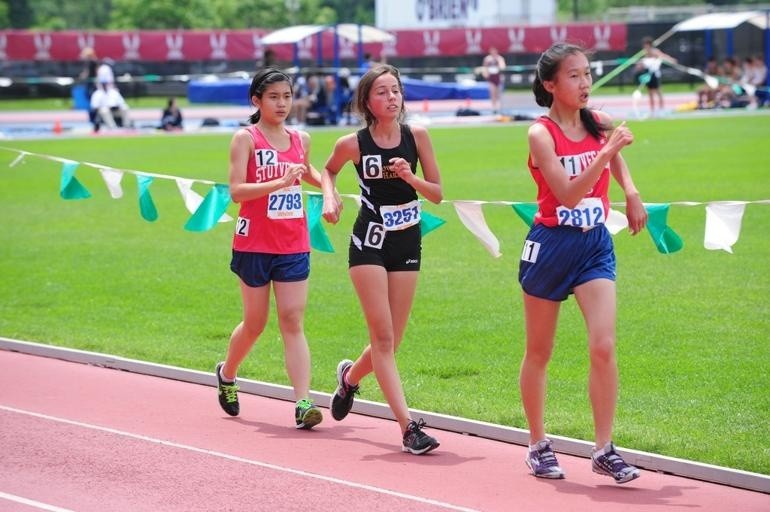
[401,418,440,456]
[295,399,323,430]
[590,441,641,484]
[215,362,240,416]
[330,359,360,420]
[525,438,565,480]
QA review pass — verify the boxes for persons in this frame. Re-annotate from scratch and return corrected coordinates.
[483,46,506,114]
[78,47,106,133]
[215,66,322,430]
[693,52,768,110]
[161,97,183,133]
[518,42,648,484]
[637,36,678,117]
[321,64,443,456]
[265,49,388,129]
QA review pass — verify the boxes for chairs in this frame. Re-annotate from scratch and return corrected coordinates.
[86,81,122,131]
